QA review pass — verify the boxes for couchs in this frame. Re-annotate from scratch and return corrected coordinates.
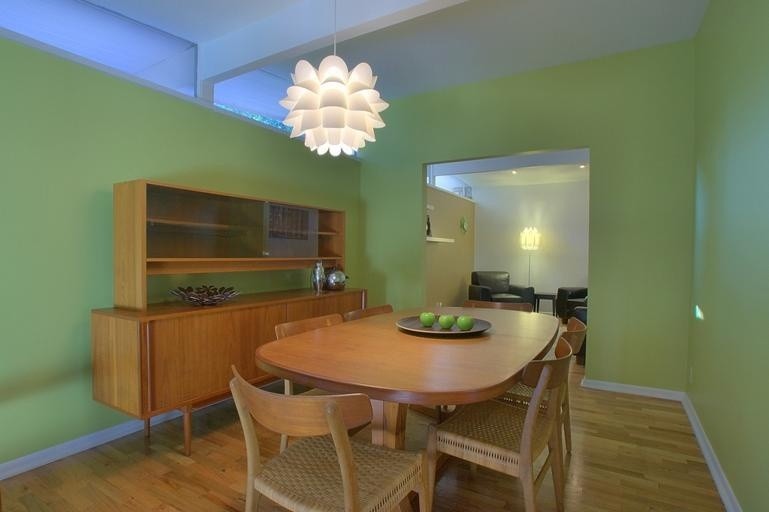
[469,271,535,312]
[557,285,587,323]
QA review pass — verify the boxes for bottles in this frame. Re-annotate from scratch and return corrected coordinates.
[310,260,325,296]
[427,215,430,236]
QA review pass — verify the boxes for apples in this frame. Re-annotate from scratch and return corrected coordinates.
[420,312,435,327]
[439,314,455,329]
[457,315,474,330]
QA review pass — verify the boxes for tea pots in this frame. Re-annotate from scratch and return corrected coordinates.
[322,265,350,290]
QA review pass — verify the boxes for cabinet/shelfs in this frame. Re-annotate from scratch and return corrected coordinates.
[113,177,345,311]
[88,288,368,457]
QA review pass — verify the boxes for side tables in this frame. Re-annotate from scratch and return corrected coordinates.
[534,292,557,315]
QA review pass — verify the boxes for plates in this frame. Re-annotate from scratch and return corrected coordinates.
[394,314,491,336]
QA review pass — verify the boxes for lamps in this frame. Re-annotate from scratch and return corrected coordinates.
[519,227,540,286]
[278,1,390,158]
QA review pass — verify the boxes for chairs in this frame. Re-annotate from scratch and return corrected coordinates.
[273,313,343,454]
[228,363,430,511]
[428,337,569,512]
[476,317,586,455]
[346,304,391,322]
